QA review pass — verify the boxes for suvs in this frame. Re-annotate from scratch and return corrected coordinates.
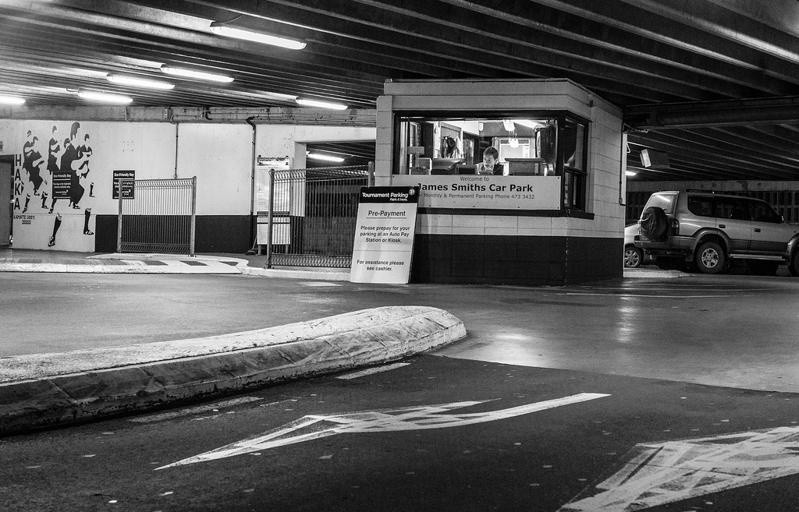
[633,192,799,278]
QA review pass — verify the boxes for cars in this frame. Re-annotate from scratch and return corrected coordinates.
[624,221,695,268]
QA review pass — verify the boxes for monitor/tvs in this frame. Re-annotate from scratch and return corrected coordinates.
[535,124,555,160]
[431,158,461,174]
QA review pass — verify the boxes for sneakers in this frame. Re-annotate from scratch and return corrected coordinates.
[73,205,80,209]
[48,237,56,246]
[22,207,27,213]
[41,205,48,209]
[49,207,53,214]
[83,229,93,235]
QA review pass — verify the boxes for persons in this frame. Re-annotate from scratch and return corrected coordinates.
[22,120,94,248]
[473,146,500,175]
